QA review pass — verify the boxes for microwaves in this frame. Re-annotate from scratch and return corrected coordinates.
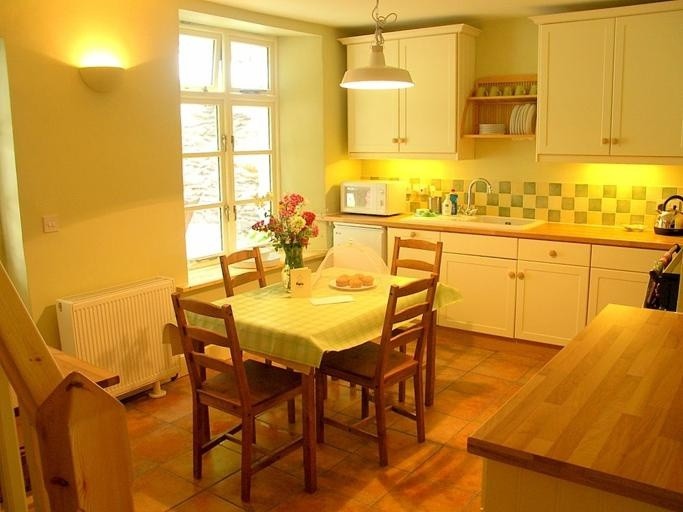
[338,179,407,216]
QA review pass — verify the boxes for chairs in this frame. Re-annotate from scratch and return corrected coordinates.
[313,271,438,467]
[171,286,302,503]
[218,246,271,366]
[350,236,442,403]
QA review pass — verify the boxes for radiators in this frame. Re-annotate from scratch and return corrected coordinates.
[54,275,181,399]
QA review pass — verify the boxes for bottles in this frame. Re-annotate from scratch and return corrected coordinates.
[441,189,458,215]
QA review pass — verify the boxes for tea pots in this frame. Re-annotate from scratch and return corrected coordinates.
[653,195,683,235]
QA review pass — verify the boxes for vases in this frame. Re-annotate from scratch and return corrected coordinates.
[281,244,306,297]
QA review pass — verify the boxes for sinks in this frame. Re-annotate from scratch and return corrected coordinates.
[463,214,545,232]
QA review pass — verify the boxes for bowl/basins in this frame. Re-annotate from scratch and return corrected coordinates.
[416,209,429,217]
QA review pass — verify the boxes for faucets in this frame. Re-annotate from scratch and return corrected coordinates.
[459,177,493,216]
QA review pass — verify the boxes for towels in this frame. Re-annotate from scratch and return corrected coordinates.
[643,250,672,308]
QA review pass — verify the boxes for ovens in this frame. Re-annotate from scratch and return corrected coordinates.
[643,243,683,313]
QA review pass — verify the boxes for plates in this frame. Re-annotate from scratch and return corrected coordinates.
[506,103,535,136]
[477,123,508,137]
[624,224,647,232]
[328,278,376,290]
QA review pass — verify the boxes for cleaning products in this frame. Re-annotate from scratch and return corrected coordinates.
[450,189,458,215]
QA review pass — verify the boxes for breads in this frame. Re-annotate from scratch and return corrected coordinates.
[336,273,374,289]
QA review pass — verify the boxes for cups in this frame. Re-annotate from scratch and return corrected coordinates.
[431,197,442,213]
[472,84,539,98]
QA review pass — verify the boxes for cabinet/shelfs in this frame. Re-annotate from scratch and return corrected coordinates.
[332,222,387,264]
[586,244,676,327]
[535,10,682,159]
[387,227,440,327]
[336,32,483,162]
[440,232,591,347]
[460,73,537,138]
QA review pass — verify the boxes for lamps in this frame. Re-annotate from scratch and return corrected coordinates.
[79,66,126,93]
[339,1,414,90]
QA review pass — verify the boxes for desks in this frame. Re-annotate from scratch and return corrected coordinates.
[182,266,465,493]
[467,303,683,507]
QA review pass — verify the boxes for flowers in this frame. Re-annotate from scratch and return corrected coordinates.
[250,190,320,286]
